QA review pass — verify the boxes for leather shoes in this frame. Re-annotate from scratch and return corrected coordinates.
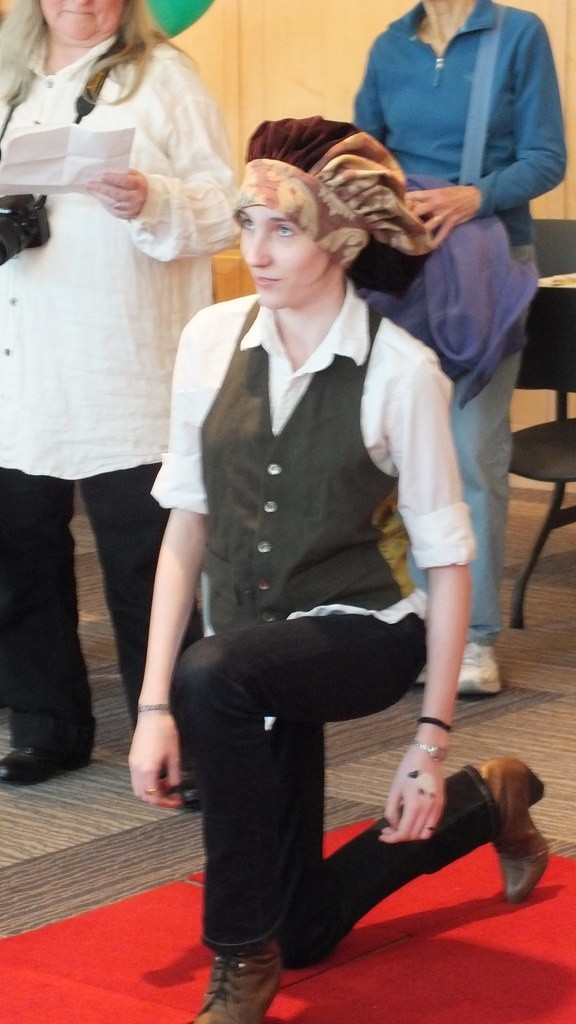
[192,938,283,1024]
[159,769,200,803]
[0,740,95,783]
[477,756,549,905]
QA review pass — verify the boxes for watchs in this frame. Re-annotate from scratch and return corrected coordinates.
[412,740,447,764]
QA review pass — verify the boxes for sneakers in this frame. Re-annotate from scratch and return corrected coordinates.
[454,642,501,695]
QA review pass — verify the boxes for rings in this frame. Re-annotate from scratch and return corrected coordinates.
[425,824,437,833]
[113,200,121,210]
[145,787,162,795]
[432,215,445,224]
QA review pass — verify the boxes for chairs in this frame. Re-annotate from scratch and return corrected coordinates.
[508,218,575,630]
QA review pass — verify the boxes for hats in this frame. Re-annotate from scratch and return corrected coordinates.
[230,115,434,273]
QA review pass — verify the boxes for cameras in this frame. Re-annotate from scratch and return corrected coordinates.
[0,194,51,266]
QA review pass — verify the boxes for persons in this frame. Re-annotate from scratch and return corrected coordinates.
[350,1,568,698]
[2,1,247,785]
[126,112,545,1024]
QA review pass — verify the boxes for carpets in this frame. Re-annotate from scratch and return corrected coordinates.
[2,817,575,1022]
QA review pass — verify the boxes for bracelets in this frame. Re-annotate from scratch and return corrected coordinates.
[416,716,453,735]
